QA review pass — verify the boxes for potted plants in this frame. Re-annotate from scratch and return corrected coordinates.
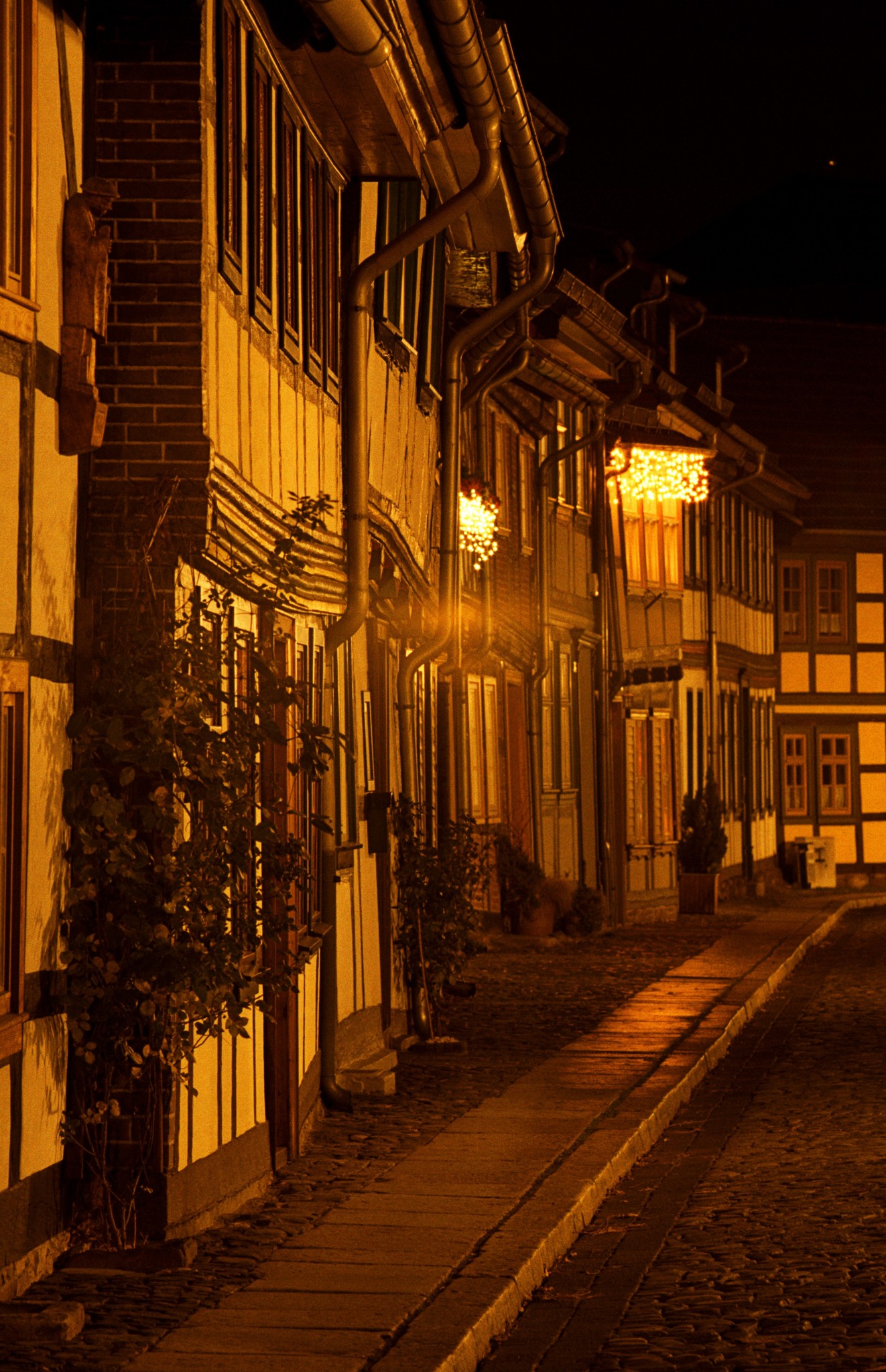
[675,765,729,913]
